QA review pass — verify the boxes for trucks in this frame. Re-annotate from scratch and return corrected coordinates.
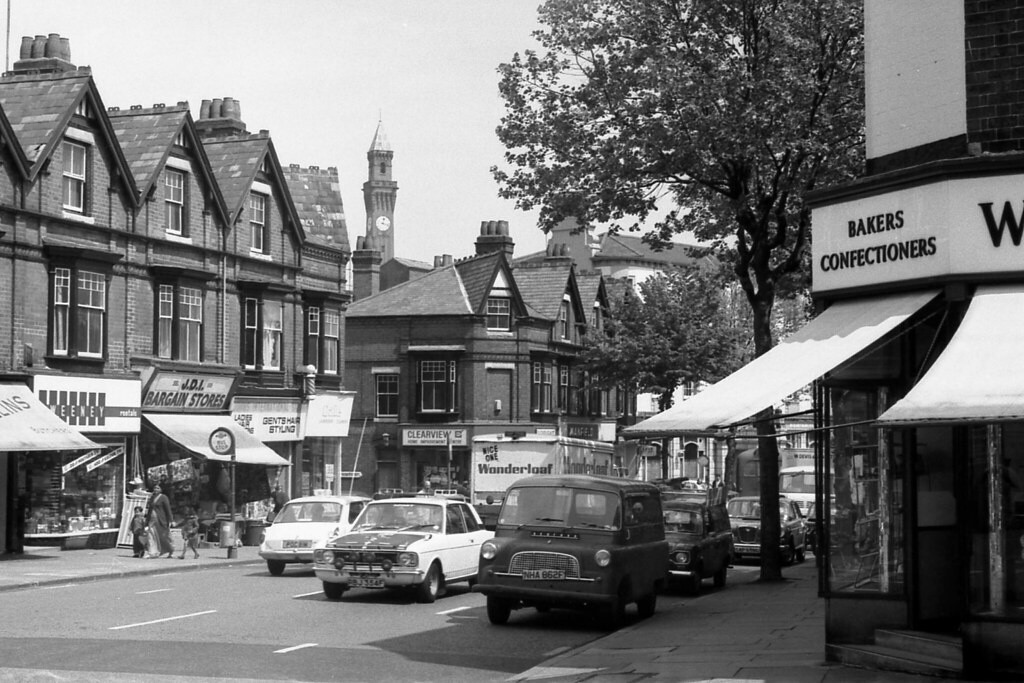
[736,446,816,497]
[469,432,629,530]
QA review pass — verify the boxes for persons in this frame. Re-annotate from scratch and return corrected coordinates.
[434,510,442,526]
[172,504,201,557]
[128,506,149,559]
[418,480,434,499]
[270,484,289,519]
[712,474,724,488]
[695,478,706,492]
[143,483,175,559]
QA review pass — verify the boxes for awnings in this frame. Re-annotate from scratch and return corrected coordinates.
[0,380,108,452]
[146,412,293,469]
[876,275,1024,424]
[620,288,941,436]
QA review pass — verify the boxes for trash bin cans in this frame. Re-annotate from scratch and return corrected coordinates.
[219,519,235,548]
[245,520,264,546]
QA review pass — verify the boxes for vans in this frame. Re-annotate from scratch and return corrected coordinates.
[468,474,672,632]
[777,466,835,552]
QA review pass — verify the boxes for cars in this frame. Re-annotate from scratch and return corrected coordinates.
[372,492,415,519]
[311,493,496,604]
[659,486,735,596]
[725,496,809,565]
[406,493,469,526]
[258,494,383,576]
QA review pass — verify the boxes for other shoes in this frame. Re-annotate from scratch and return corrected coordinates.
[194,553,199,558]
[179,555,184,559]
